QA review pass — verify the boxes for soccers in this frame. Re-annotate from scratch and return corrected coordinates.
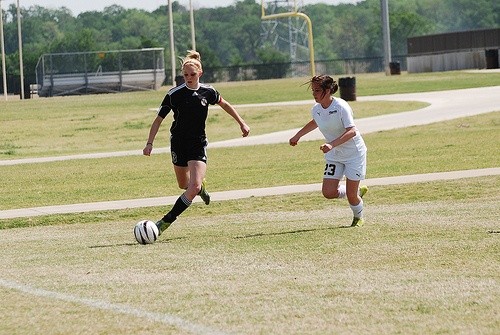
[133,220,159,244]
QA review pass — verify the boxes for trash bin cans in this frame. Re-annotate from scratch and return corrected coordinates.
[338,76,357,102]
[389,61,401,75]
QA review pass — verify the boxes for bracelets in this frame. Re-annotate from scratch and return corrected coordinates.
[147,143,152,145]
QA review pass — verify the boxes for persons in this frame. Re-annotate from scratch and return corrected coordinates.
[143,50,250,235]
[289,74,368,228]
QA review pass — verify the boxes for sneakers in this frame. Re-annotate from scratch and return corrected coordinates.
[358,185,370,199]
[197,177,211,205]
[352,217,363,227]
[155,219,173,237]
